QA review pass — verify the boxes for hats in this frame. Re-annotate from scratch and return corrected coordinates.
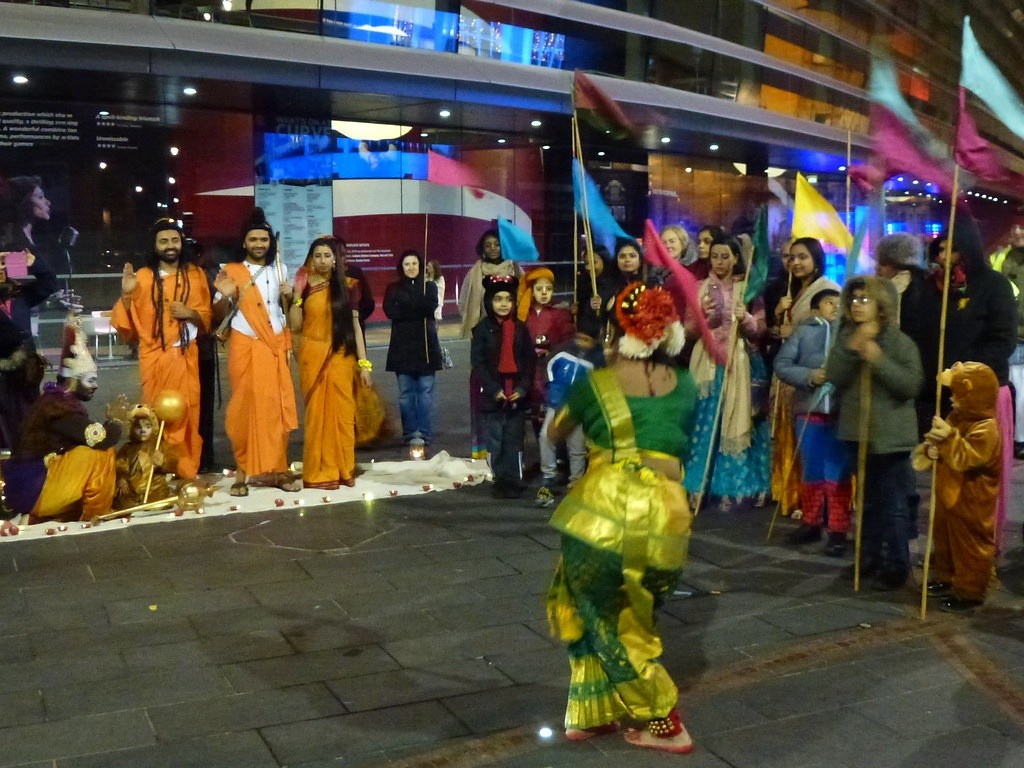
[877,233,927,268]
[22,352,46,400]
[615,281,685,359]
[59,314,98,378]
[482,275,520,323]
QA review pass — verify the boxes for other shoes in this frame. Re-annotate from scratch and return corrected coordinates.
[840,562,907,593]
[541,477,575,490]
[687,491,766,513]
[785,526,819,544]
[230,482,247,496]
[941,597,984,612]
[279,478,301,492]
[919,582,953,596]
[403,435,431,445]
[826,536,847,556]
[494,481,520,498]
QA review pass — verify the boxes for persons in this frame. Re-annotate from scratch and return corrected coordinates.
[546,282,699,753]
[459,217,1024,614]
[0,207,445,527]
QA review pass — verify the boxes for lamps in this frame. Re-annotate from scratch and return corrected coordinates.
[409,427,426,460]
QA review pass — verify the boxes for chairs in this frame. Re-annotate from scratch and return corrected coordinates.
[30,315,44,357]
[91,310,118,360]
[80,316,115,361]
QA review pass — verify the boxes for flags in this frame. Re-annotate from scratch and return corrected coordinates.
[427,67,770,372]
[792,14,1023,251]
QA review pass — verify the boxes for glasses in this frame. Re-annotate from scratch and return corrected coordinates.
[851,296,873,304]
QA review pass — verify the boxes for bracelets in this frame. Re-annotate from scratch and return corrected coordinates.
[292,298,302,306]
[357,359,372,372]
